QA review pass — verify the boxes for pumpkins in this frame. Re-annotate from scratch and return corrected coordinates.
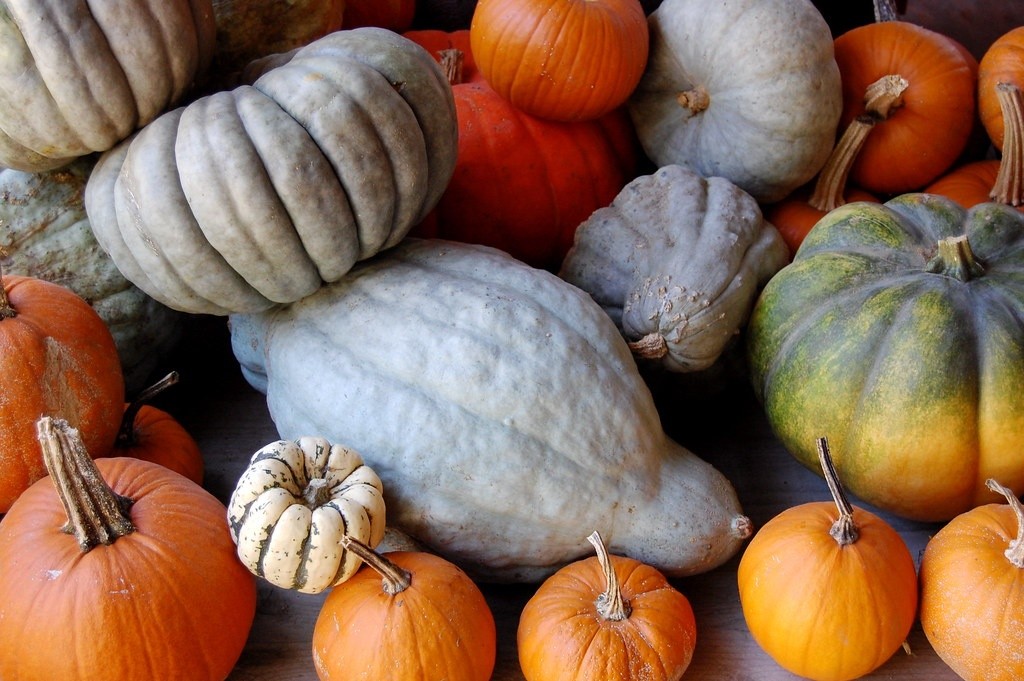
[0,0,1024,681]
